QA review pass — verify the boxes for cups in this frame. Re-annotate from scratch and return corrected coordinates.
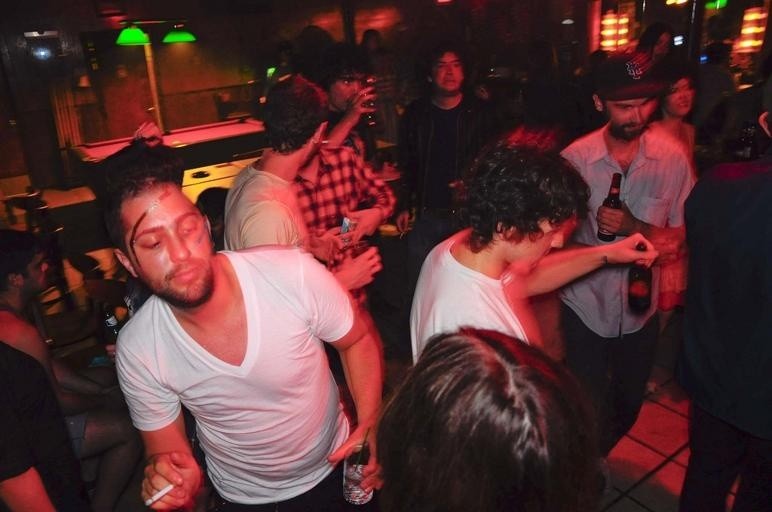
[340,239,370,258]
[357,92,384,130]
[342,445,375,506]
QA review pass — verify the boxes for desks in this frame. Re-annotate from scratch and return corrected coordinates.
[68,117,267,183]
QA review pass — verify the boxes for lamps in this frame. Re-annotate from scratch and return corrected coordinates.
[159,18,198,44]
[115,20,153,46]
[600,10,629,52]
[736,7,768,52]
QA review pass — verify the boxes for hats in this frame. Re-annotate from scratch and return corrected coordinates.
[593,52,671,102]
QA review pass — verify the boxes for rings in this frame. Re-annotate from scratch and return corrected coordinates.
[112,351,116,359]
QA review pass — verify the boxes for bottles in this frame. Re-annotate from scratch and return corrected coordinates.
[104,311,122,342]
[597,172,622,242]
[743,120,759,160]
[627,242,653,315]
[734,121,749,162]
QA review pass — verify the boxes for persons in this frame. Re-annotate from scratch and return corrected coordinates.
[411,133,658,366]
[560,56,697,452]
[377,328,610,511]
[224,30,583,365]
[638,25,770,178]
[0,230,144,511]
[676,88,772,512]
[105,144,214,510]
[107,168,386,512]
